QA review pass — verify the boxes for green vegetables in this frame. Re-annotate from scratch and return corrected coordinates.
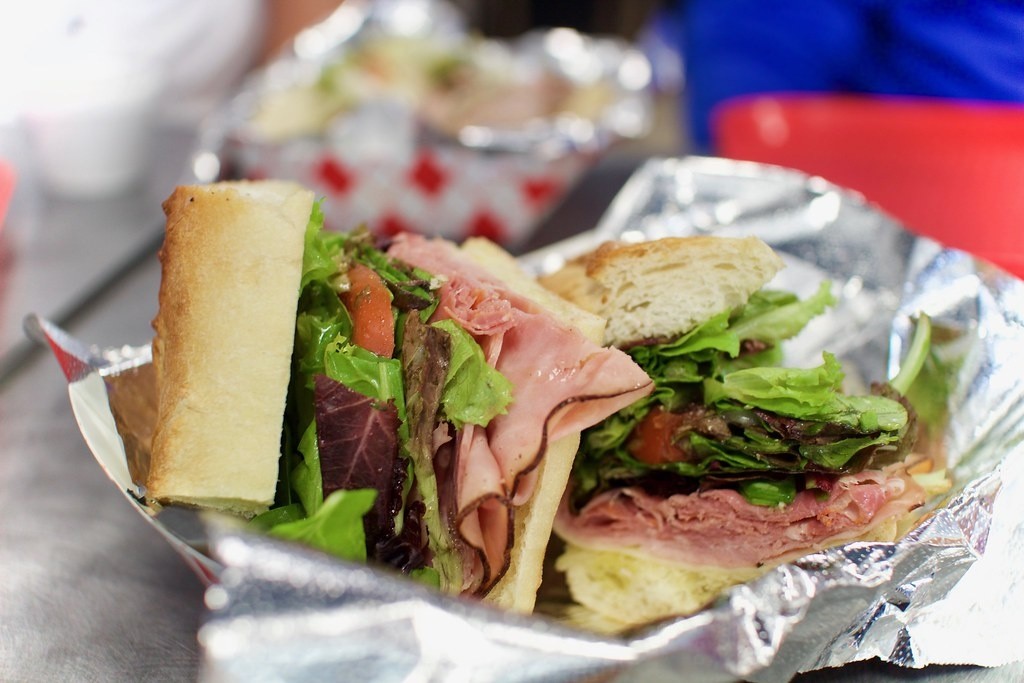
[247,195,972,607]
